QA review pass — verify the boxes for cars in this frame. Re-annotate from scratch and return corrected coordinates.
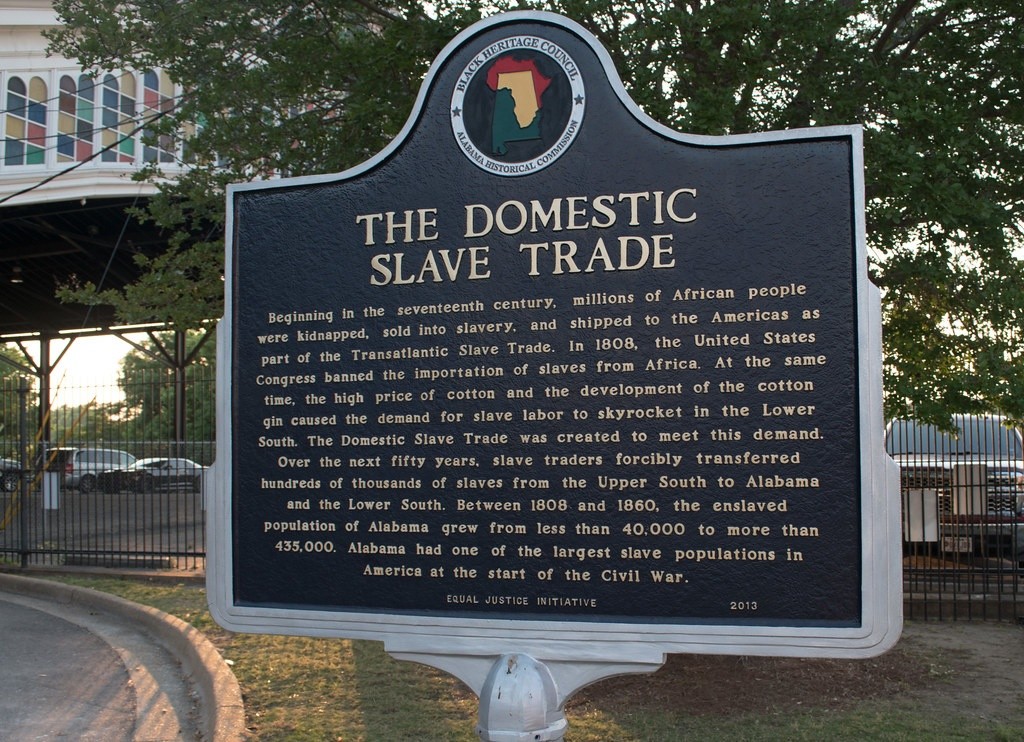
[99,457,210,495]
[0,457,36,493]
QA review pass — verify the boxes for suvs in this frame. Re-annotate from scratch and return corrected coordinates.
[882,413,1024,570]
[32,447,140,494]
